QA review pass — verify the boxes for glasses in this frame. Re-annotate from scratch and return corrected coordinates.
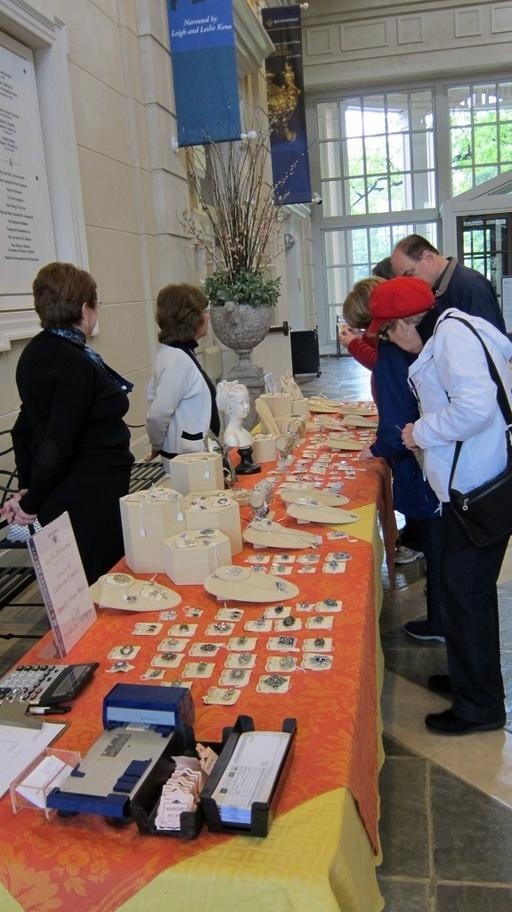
[402,259,419,277]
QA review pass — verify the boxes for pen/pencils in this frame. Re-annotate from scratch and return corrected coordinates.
[27,705,71,714]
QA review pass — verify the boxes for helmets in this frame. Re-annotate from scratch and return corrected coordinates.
[376,325,391,341]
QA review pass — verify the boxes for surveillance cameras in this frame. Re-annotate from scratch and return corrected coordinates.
[312,192,322,205]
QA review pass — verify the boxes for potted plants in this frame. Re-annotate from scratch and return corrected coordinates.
[201,269,282,349]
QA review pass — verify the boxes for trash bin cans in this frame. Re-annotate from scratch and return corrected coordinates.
[291,331,322,378]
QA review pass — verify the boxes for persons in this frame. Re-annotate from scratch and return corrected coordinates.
[143,282,221,478]
[0,261,135,585]
[335,255,429,567]
[390,234,507,336]
[367,276,512,736]
[341,276,447,643]
[216,377,255,448]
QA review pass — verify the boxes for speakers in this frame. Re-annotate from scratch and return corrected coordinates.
[290,330,321,378]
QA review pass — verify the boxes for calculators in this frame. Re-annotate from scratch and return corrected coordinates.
[0,662,99,706]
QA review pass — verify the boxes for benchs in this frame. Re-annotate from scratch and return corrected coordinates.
[0,423,167,639]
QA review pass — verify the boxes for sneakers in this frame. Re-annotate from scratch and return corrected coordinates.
[429,674,451,696]
[404,621,445,642]
[394,545,424,564]
[425,709,506,735]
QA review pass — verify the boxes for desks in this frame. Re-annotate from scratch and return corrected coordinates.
[0,399,385,912]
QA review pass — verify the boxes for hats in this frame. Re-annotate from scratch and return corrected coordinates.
[367,276,435,334]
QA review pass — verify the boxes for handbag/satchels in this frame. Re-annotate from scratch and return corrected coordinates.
[448,465,511,547]
